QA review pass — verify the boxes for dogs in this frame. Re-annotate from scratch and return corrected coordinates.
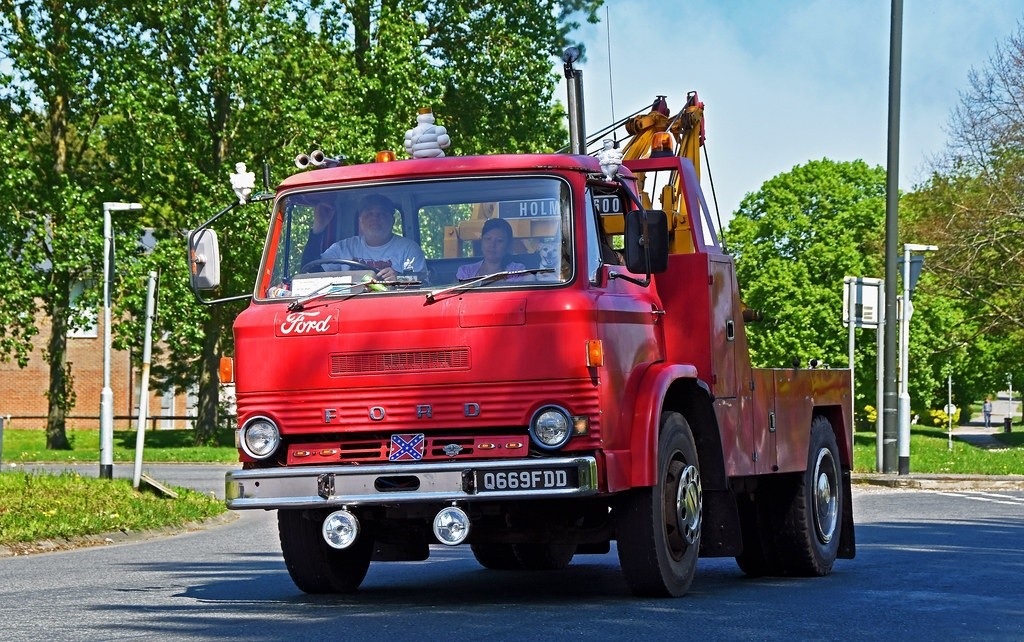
[536,241,571,282]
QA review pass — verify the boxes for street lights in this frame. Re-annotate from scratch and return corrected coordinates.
[99,200,145,482]
[1006,382,1012,418]
[897,244,939,474]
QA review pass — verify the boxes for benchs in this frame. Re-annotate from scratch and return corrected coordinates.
[426,253,541,285]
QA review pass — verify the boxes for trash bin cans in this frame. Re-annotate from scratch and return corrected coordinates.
[1004,418,1012,433]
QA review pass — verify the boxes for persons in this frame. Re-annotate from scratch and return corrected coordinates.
[454,217,528,284]
[982,397,992,426]
[307,194,429,288]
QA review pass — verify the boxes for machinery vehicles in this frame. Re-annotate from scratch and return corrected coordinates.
[184,48,857,599]
